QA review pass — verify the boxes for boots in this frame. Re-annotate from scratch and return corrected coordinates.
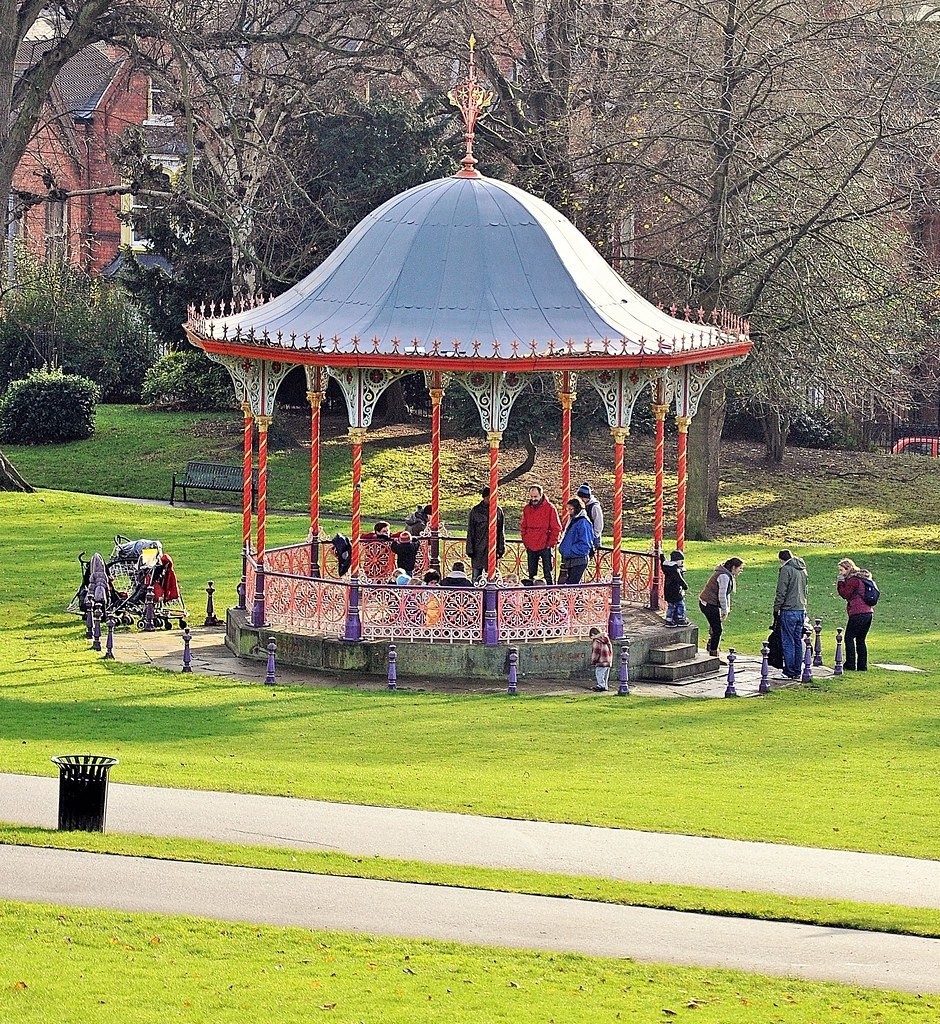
[710,650,727,665]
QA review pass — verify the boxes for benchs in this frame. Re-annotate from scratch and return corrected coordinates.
[169,461,271,513]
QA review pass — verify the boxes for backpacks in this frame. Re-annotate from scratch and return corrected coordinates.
[849,576,880,606]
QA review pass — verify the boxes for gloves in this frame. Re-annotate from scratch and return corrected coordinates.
[773,610,779,618]
[377,534,389,540]
[468,554,475,558]
[497,554,503,559]
[837,575,844,580]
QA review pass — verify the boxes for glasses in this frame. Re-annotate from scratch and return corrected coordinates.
[566,507,576,511]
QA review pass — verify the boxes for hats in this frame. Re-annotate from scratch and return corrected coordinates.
[670,551,685,561]
[577,482,591,498]
[482,487,490,498]
[397,575,411,585]
[399,531,412,543]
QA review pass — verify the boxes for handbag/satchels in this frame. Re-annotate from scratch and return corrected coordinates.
[767,615,783,669]
[589,545,596,559]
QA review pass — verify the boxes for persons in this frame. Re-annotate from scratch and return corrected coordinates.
[577,482,604,559]
[588,628,614,692]
[699,558,744,665]
[557,498,594,584]
[662,551,692,628]
[361,521,402,576]
[390,531,419,578]
[403,505,433,546]
[385,560,569,638]
[465,487,505,588]
[836,557,875,672]
[520,484,562,585]
[773,549,809,680]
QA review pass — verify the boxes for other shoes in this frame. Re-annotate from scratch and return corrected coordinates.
[591,685,607,691]
[771,672,801,680]
[665,620,691,627]
